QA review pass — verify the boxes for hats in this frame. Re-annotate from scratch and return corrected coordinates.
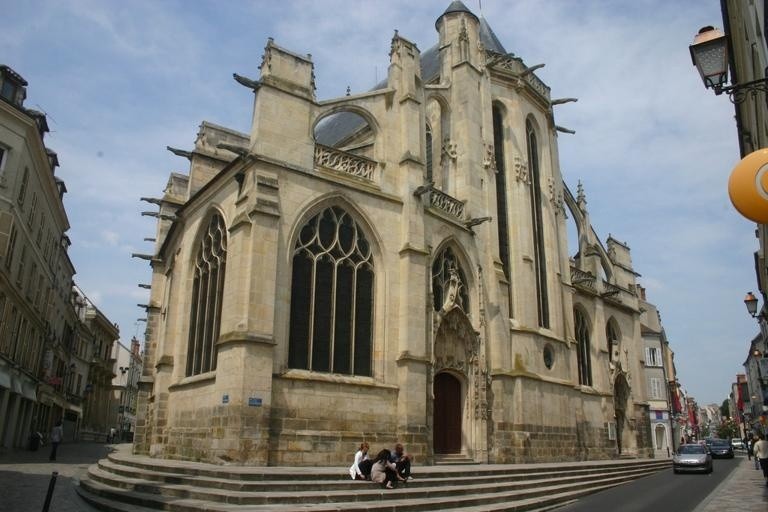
[673,438,744,473]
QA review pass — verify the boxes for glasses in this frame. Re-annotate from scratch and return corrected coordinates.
[744,291,768,323]
[688,26,768,103]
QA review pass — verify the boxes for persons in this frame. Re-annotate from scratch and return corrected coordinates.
[370,448,407,489]
[390,443,414,479]
[349,442,372,480]
[753,433,768,487]
[49,419,64,460]
